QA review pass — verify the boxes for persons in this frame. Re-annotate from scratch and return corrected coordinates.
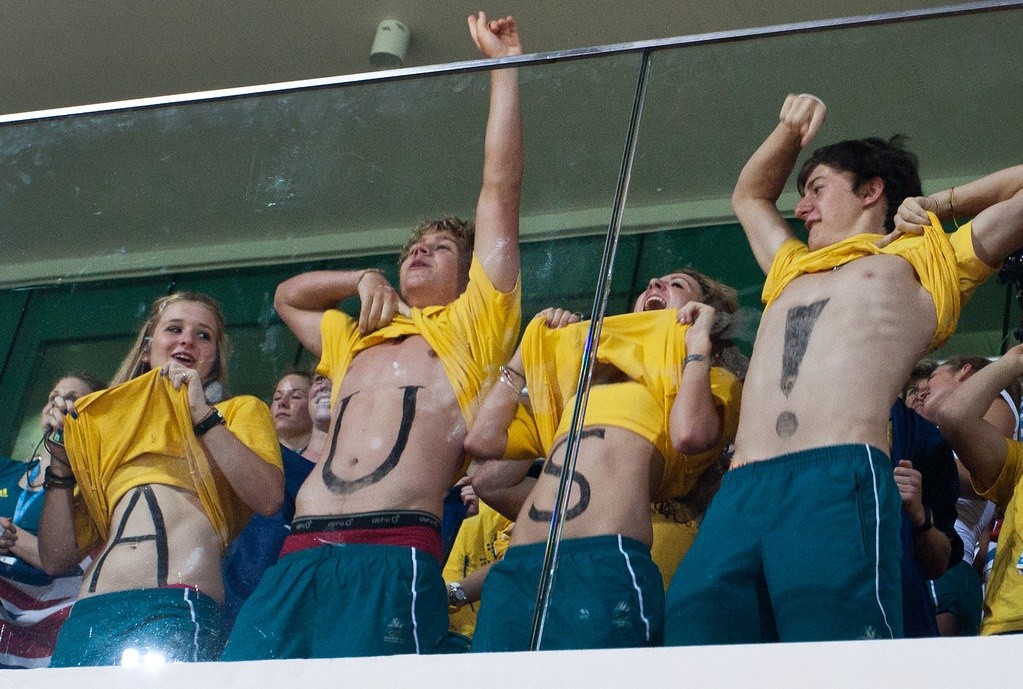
[282,364,336,514]
[0,370,113,669]
[266,365,315,470]
[37,289,287,667]
[463,269,750,655]
[663,91,1022,645]
[215,8,526,661]
[431,339,1023,653]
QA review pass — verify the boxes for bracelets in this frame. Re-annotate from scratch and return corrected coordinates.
[948,185,961,232]
[42,466,76,491]
[195,407,212,426]
[355,268,385,293]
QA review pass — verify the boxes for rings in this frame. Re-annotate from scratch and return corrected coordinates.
[49,407,54,417]
[178,367,184,373]
[0,525,5,538]
[182,373,186,382]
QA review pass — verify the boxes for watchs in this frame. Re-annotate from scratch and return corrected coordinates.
[193,406,226,438]
